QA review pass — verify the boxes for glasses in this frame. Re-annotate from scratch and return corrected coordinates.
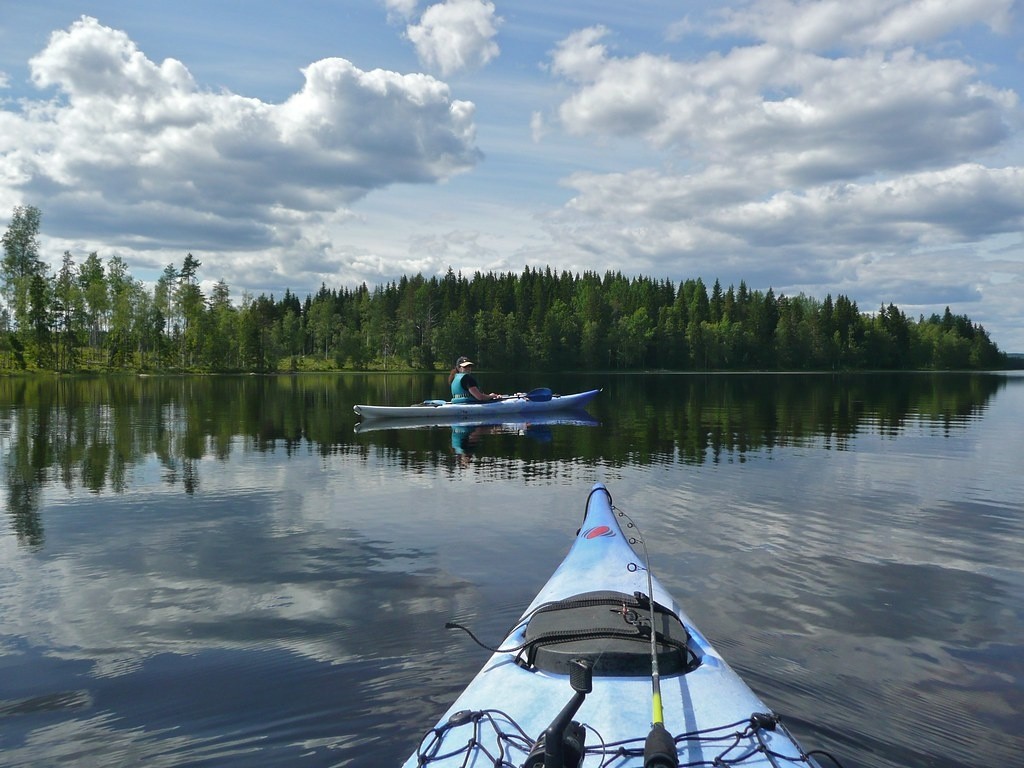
[461,365,471,368]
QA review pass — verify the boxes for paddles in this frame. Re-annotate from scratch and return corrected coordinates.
[423,388,552,405]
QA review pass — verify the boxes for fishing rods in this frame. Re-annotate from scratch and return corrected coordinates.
[610,504,670,768]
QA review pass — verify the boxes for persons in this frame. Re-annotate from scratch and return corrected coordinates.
[449,356,497,400]
[449,426,482,468]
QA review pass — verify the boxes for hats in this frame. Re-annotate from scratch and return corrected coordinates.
[456,356,474,368]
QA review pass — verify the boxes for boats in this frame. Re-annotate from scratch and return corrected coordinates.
[352,387,604,420]
[353,408,605,435]
